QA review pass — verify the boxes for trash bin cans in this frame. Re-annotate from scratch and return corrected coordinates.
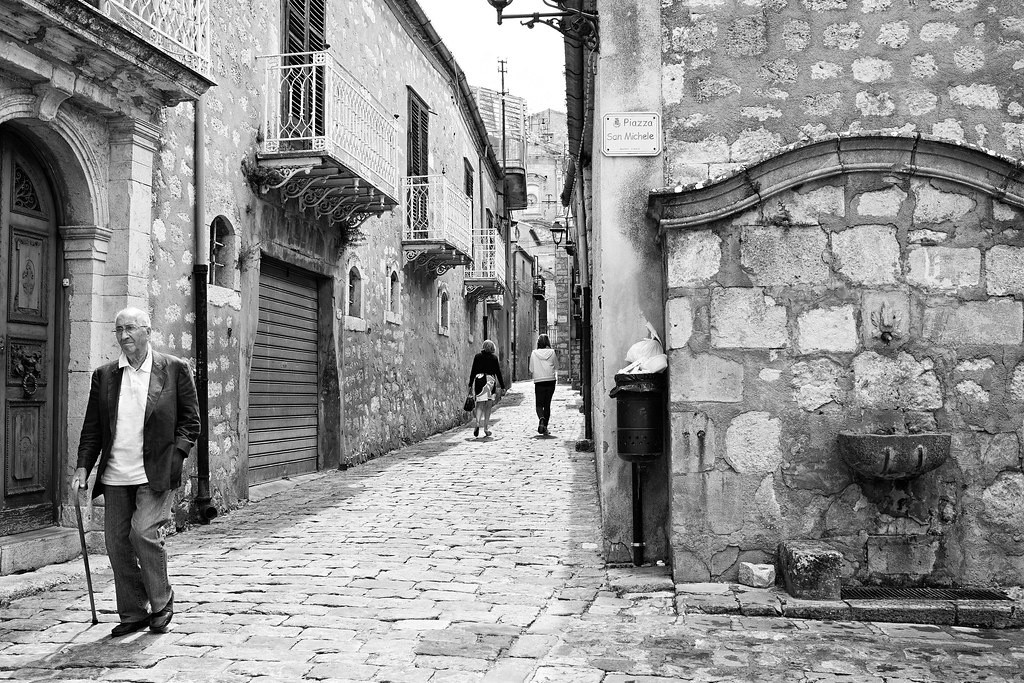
[615,373,665,463]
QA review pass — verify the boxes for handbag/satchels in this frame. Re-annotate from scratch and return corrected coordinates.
[464,394,475,411]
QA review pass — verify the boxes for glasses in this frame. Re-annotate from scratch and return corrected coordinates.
[112,324,147,334]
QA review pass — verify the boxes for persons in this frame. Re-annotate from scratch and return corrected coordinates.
[529,333,559,435]
[71,307,202,636]
[469,340,505,437]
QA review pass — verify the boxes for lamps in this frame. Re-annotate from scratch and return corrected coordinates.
[549,221,576,249]
[487,0,600,53]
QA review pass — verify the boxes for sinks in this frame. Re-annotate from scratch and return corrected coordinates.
[838,432,952,480]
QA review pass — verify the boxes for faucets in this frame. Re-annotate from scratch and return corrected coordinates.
[881,332,893,346]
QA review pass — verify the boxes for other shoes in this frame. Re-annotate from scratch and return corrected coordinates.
[474,426,479,437]
[538,420,550,435]
[484,431,491,436]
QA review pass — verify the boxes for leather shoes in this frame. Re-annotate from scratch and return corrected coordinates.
[111,613,150,636]
[150,590,174,632]
